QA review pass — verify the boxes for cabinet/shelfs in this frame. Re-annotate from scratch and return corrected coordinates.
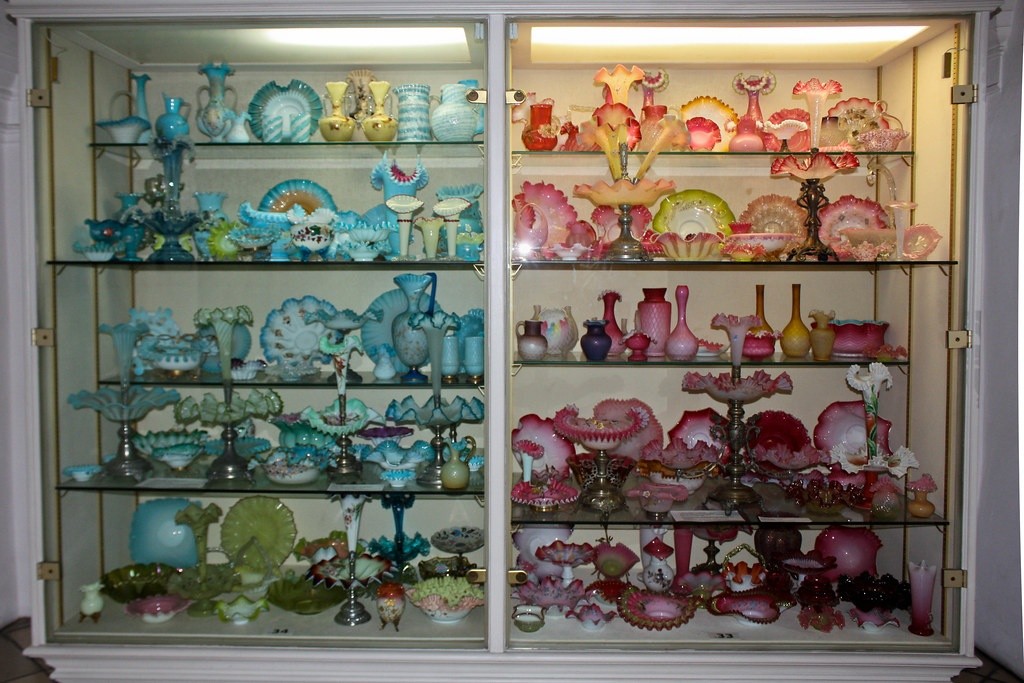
[4,2,1006,683]
